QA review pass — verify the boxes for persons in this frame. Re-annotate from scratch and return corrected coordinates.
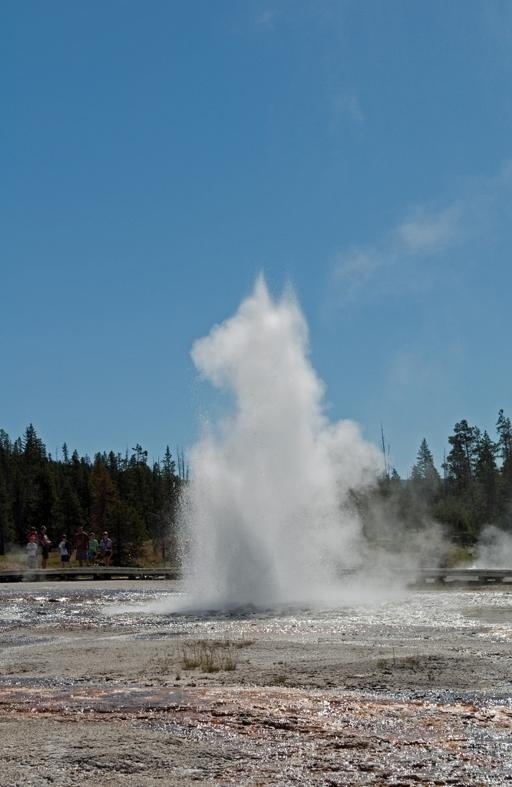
[24,525,113,569]
